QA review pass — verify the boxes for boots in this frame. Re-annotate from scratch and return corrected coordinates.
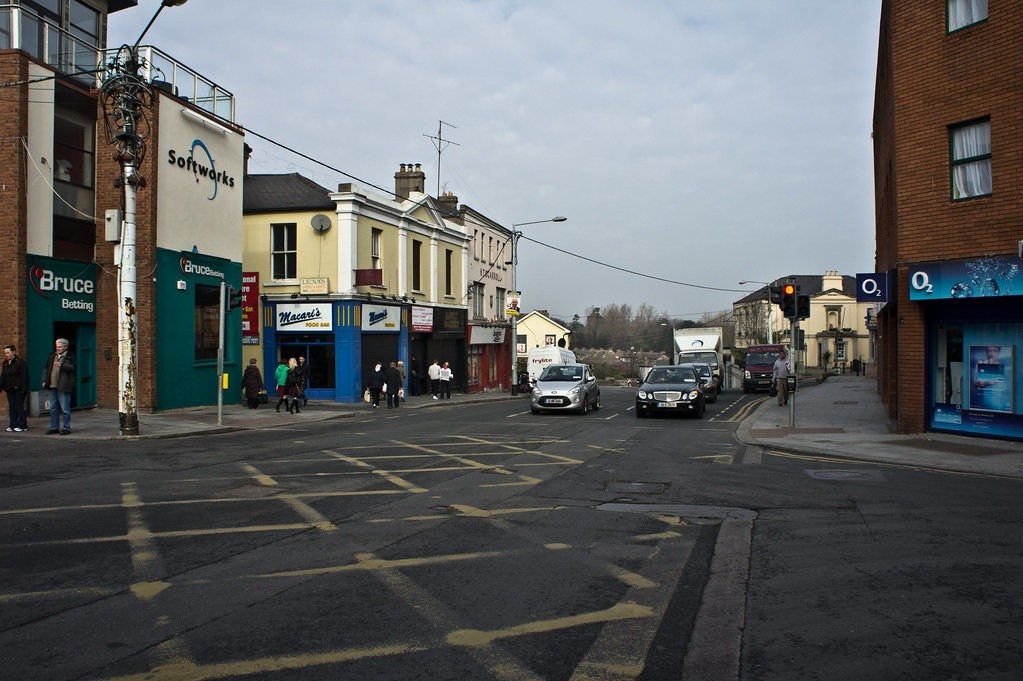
[285,398,290,411]
[276,398,284,412]
[295,400,300,413]
[290,400,297,414]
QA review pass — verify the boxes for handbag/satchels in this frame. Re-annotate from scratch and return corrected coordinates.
[297,387,304,398]
[769,382,777,397]
[398,388,404,399]
[256,389,268,404]
[364,389,370,402]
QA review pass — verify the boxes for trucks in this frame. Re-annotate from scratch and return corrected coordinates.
[673,325,727,393]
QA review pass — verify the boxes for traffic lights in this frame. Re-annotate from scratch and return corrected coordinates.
[796,295,812,321]
[769,284,784,310]
[781,282,795,319]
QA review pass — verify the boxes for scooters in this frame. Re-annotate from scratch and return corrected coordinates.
[520,371,529,394]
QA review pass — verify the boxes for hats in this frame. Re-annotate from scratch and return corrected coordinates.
[398,361,403,367]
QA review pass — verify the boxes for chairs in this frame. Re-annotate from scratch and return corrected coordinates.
[683,373,691,379]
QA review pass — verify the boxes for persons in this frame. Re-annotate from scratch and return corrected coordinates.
[0,345,29,431]
[428,360,453,400]
[274,356,310,414]
[509,299,520,313]
[772,350,789,405]
[370,361,406,408]
[42,339,75,436]
[242,359,265,409]
[974,347,1001,388]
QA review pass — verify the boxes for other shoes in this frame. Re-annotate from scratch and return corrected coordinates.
[433,395,438,400]
[304,401,307,406]
[46,429,59,434]
[784,400,788,405]
[60,430,70,435]
[778,401,782,406]
[377,404,380,408]
[372,404,376,408]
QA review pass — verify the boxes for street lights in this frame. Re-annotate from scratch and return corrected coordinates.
[112,0,192,434]
[509,217,568,397]
[739,281,774,347]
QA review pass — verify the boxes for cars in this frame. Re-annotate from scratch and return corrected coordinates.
[687,363,719,404]
[528,363,602,415]
[635,364,708,420]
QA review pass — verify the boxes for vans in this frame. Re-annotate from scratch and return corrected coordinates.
[527,347,581,394]
[743,342,789,393]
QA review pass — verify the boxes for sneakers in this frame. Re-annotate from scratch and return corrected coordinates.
[5,428,13,432]
[14,427,28,432]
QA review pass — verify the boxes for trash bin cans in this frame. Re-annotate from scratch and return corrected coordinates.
[853,359,860,371]
